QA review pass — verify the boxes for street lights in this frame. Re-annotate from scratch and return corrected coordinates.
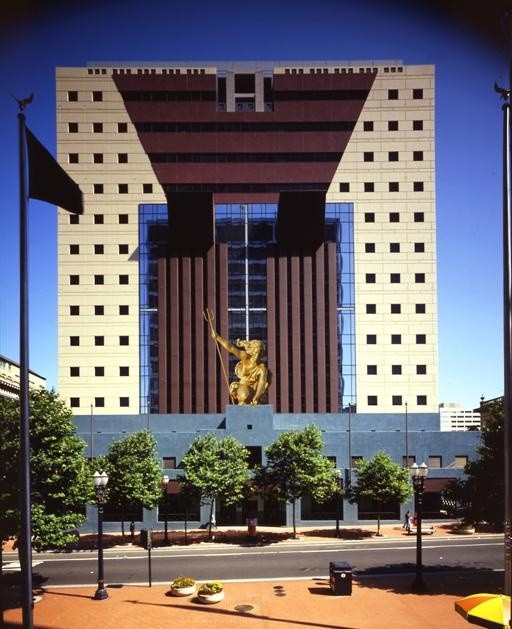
[410,462,428,585]
[91,471,109,599]
[335,470,341,537]
[163,475,169,543]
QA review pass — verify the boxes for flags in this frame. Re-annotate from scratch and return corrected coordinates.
[23,122,86,216]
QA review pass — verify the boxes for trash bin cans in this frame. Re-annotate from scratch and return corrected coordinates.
[329,562,352,595]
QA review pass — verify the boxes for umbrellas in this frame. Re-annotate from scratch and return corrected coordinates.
[452,593,512,629]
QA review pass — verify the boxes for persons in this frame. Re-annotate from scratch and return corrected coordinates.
[211,328,273,407]
[403,510,410,530]
[407,514,413,534]
[412,512,418,526]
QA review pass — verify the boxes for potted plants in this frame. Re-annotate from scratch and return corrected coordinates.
[171,577,196,596]
[198,583,224,604]
